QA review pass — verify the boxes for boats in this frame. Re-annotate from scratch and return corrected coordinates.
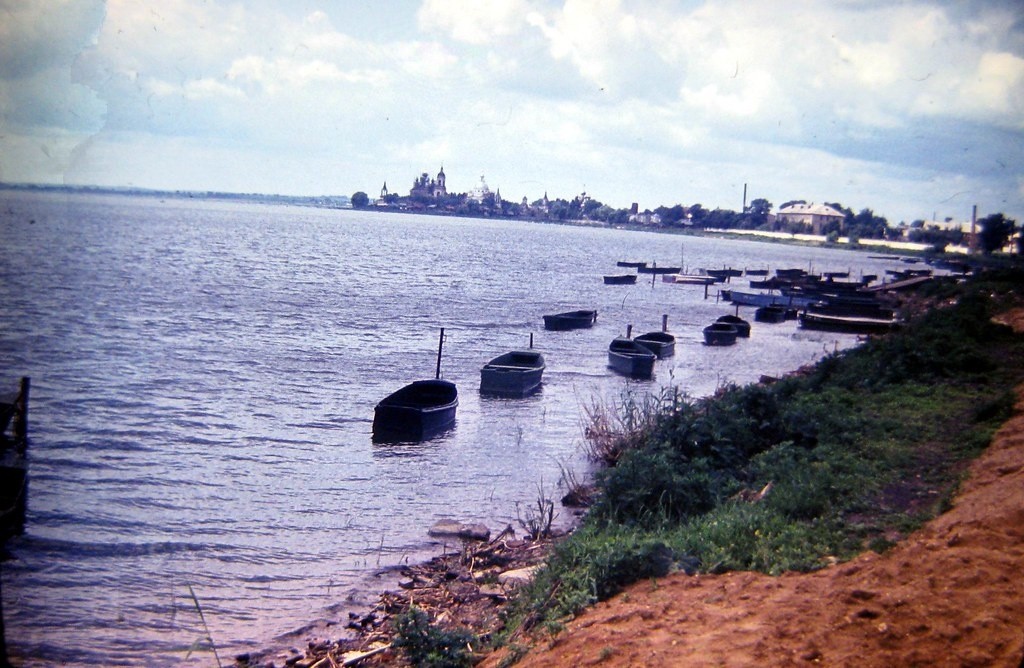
[714,313,752,338]
[711,273,727,283]
[719,267,934,306]
[607,323,658,376]
[662,243,717,285]
[541,309,599,332]
[756,307,786,322]
[616,261,648,267]
[632,313,677,357]
[636,267,682,273]
[476,332,547,396]
[795,310,900,333]
[770,301,798,320]
[603,274,638,285]
[370,326,459,440]
[705,267,743,277]
[745,266,765,276]
[701,320,739,347]
[0,375,33,557]
